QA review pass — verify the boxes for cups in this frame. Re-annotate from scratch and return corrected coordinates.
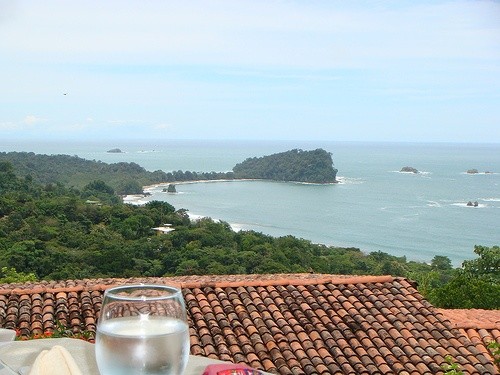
[95,283,190,375]
